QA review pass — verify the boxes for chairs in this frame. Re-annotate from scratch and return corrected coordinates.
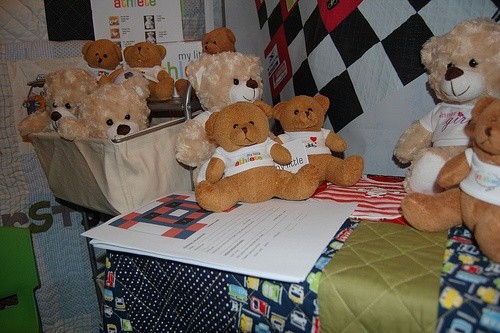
[0,226,42,332]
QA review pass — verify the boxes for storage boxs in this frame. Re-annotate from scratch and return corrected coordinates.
[28,108,204,216]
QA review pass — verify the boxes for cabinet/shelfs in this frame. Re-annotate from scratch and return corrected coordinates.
[54,196,113,321]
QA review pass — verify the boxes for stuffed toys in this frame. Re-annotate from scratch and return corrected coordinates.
[393,16,499,196]
[173,26,237,109]
[121,42,175,100]
[400,96,499,262]
[172,50,265,185]
[56,74,150,139]
[271,93,364,193]
[194,99,319,214]
[79,39,122,85]
[17,67,99,137]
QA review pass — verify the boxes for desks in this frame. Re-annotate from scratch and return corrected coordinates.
[103,173,500,332]
